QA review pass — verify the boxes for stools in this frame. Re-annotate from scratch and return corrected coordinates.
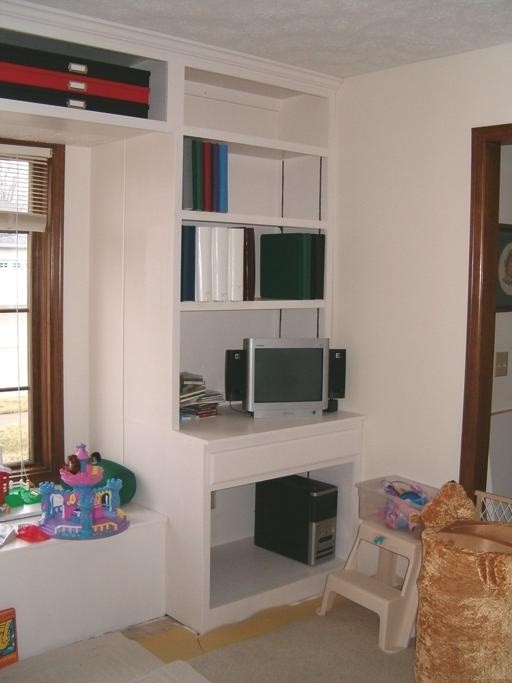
[315,522,423,656]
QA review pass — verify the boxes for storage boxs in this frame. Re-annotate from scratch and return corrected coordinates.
[355,475,440,543]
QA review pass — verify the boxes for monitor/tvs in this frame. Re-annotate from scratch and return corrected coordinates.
[242,338,329,420]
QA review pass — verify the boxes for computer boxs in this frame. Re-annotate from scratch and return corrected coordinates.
[254,474,339,567]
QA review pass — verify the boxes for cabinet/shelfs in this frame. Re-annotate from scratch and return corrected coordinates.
[0,0,367,666]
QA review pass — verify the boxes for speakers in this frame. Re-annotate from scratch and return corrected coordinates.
[328,348,346,400]
[225,350,247,401]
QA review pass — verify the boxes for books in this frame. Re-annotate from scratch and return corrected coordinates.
[1,607,19,670]
[182,135,232,215]
[180,225,324,301]
[180,371,225,421]
